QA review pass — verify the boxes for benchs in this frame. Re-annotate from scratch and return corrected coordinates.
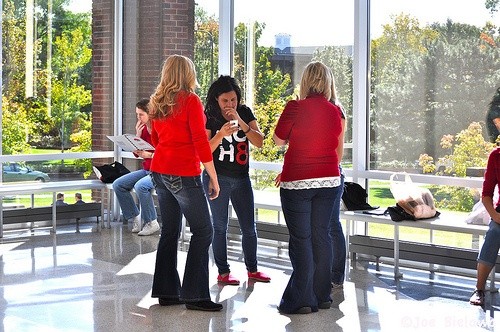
[0,180,496,293]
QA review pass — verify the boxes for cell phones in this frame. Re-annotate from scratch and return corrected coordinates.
[230,119,238,134]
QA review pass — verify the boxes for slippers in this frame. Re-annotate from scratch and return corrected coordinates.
[470,290,485,306]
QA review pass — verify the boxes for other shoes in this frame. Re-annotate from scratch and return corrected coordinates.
[248,271,272,282]
[318,301,332,308]
[217,273,241,286]
[331,282,343,290]
[131,219,144,233]
[138,218,160,236]
[185,299,224,313]
[158,296,186,305]
[296,306,318,315]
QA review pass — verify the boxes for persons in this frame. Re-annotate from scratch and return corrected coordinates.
[151,54,223,312]
[51,193,68,206]
[75,193,85,204]
[202,75,271,286]
[112,98,160,236]
[470,148,500,304]
[328,68,346,289]
[273,62,342,314]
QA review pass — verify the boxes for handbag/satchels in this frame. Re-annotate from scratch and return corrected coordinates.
[361,203,441,222]
[93,161,132,183]
[341,182,380,211]
[464,187,493,225]
[389,171,436,218]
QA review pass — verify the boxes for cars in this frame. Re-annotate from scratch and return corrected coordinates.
[2,162,51,184]
